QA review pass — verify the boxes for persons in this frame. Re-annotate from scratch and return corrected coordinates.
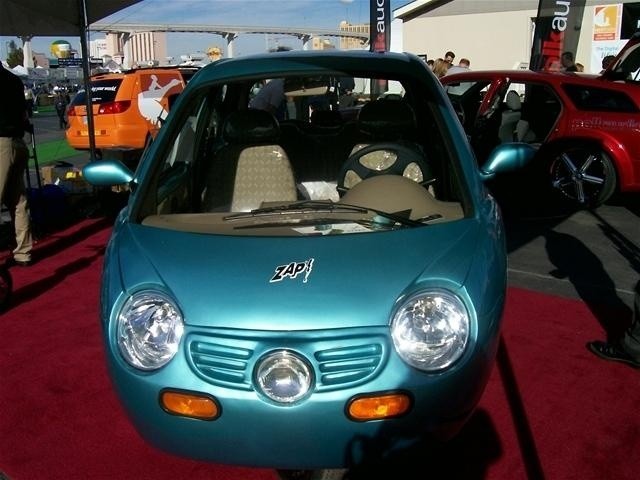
[561,52,577,72]
[427,52,477,96]
[590,280,640,366]
[576,63,585,72]
[24,84,72,130]
[600,56,615,74]
[0,62,34,267]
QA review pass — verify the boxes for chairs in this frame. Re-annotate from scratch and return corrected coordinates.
[497,89,523,143]
[201,100,435,213]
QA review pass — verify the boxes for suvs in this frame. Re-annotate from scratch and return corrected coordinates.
[64,63,203,157]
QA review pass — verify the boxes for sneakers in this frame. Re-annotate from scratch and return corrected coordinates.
[590,341,640,368]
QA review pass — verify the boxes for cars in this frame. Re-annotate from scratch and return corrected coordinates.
[439,69,640,210]
[78,47,540,480]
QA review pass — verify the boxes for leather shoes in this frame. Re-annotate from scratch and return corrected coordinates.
[6,259,30,266]
